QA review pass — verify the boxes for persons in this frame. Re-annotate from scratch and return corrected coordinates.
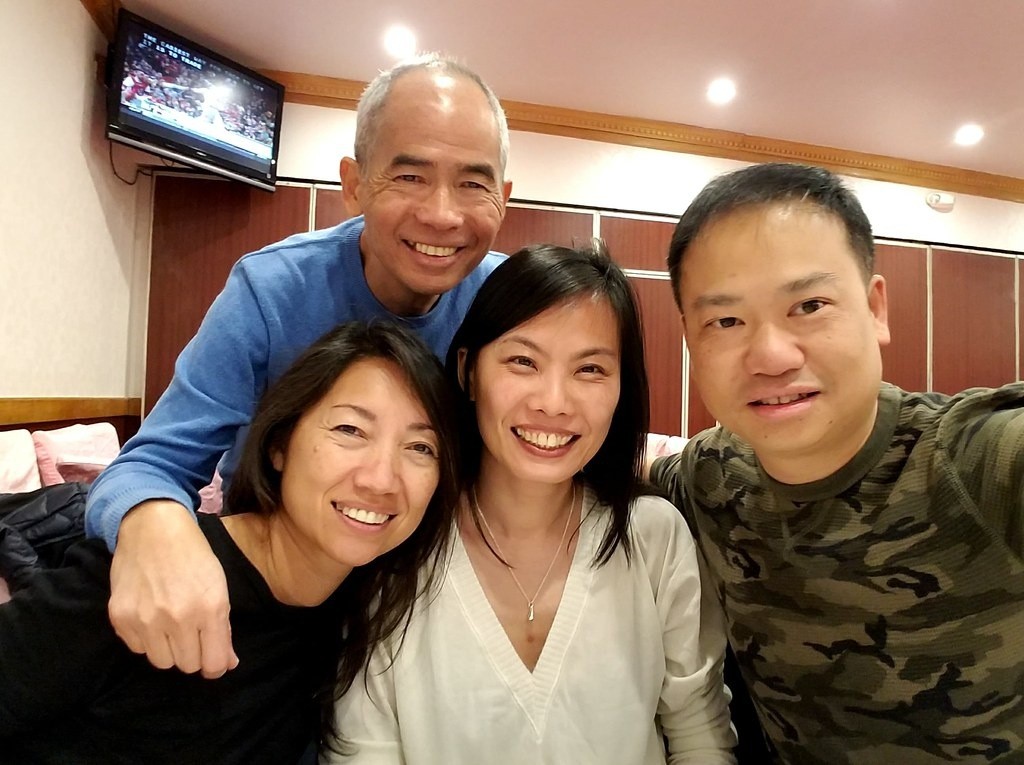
[86,53,512,680]
[635,163,1024,765]
[120,42,275,143]
[321,240,739,765]
[1,316,462,765]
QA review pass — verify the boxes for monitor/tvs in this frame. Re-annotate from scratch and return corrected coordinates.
[103,8,285,192]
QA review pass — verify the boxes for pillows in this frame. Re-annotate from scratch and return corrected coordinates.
[33,422,120,488]
[0,429,42,495]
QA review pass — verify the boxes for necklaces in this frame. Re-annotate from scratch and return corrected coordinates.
[474,488,576,621]
